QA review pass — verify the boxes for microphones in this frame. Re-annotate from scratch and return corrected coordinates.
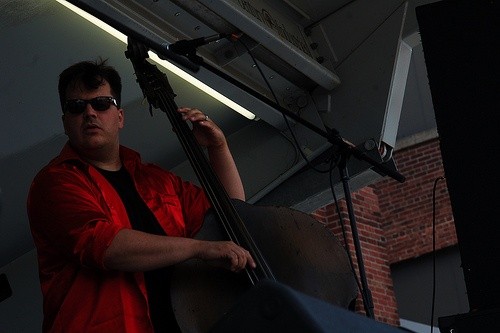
[158,33,224,60]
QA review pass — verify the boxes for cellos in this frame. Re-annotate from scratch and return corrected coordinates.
[125,38,359,333]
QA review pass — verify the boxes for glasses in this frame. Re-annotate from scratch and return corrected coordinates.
[64,96,119,114]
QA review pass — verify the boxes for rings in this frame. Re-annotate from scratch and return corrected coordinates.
[203,114,209,122]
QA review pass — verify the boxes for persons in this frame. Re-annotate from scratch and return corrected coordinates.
[27,62,256,333]
